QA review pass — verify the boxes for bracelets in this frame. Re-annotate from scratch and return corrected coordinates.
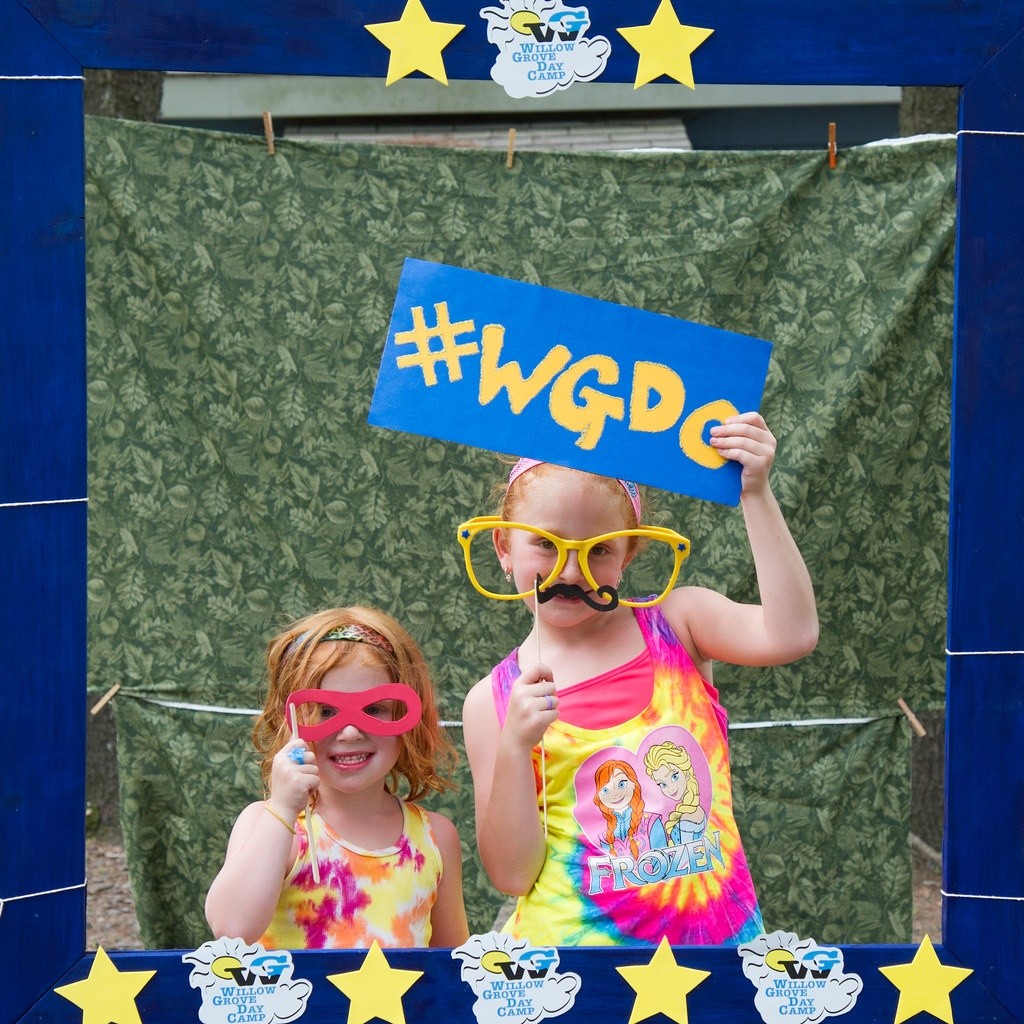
[264,804,297,836]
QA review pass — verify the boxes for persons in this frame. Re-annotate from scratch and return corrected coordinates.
[203,605,474,952]
[460,411,821,951]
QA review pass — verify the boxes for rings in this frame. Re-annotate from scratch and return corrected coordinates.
[286,746,307,766]
[544,694,552,710]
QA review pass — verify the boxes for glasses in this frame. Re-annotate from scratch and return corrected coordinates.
[280,682,423,742]
[455,513,692,610]
